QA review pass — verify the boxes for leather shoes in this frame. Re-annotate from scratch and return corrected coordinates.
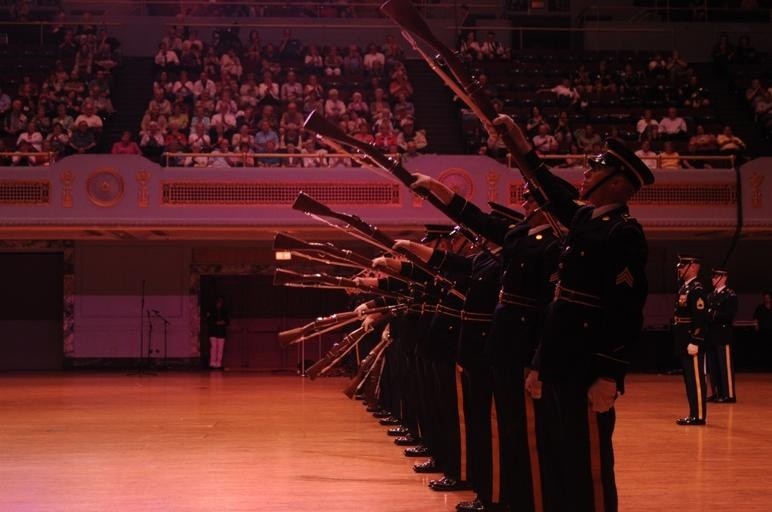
[707,396,736,403]
[676,417,704,425]
[353,392,483,510]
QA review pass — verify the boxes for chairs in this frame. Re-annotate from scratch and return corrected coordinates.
[0,46,75,99]
[484,46,772,153]
[324,75,366,90]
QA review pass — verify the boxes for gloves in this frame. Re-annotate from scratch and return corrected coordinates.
[687,343,698,355]
[352,240,411,342]
[588,377,617,413]
[407,172,430,191]
[485,114,514,138]
[524,371,538,391]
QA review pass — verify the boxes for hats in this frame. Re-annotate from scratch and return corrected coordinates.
[450,223,487,246]
[677,255,703,269]
[488,202,521,225]
[523,173,578,199]
[420,224,454,244]
[588,137,655,190]
[709,267,729,278]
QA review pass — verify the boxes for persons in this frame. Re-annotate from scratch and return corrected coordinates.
[3,10,123,168]
[706,267,736,403]
[113,130,141,156]
[349,200,524,492]
[453,22,766,167]
[492,113,654,510]
[754,294,772,351]
[410,171,579,511]
[204,298,229,370]
[454,2,477,45]
[672,253,708,425]
[137,23,426,166]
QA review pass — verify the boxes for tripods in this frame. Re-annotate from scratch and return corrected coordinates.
[127,280,181,375]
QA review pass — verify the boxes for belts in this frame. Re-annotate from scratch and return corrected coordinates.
[498,290,535,308]
[408,302,492,321]
[554,284,601,307]
[673,317,691,324]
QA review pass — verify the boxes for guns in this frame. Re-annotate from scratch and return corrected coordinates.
[271,230,435,290]
[303,315,370,382]
[272,270,416,302]
[378,0,565,238]
[302,111,498,261]
[342,335,393,401]
[278,301,409,347]
[288,189,468,295]
